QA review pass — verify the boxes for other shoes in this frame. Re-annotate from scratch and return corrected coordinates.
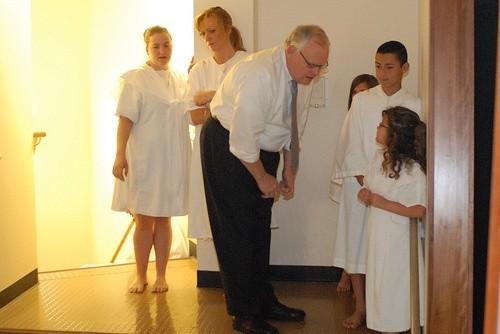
[233,315,280,334]
[261,300,307,322]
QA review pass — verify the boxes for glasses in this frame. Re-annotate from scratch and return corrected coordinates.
[379,124,388,129]
[299,52,328,69]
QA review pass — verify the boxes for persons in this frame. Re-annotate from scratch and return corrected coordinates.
[328,74,380,298]
[187,6,279,240]
[199,25,330,334]
[111,26,196,293]
[331,41,421,328]
[357,106,426,334]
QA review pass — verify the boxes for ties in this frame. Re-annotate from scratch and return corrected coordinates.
[290,80,300,179]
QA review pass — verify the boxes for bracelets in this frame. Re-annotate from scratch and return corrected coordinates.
[205,109,207,116]
